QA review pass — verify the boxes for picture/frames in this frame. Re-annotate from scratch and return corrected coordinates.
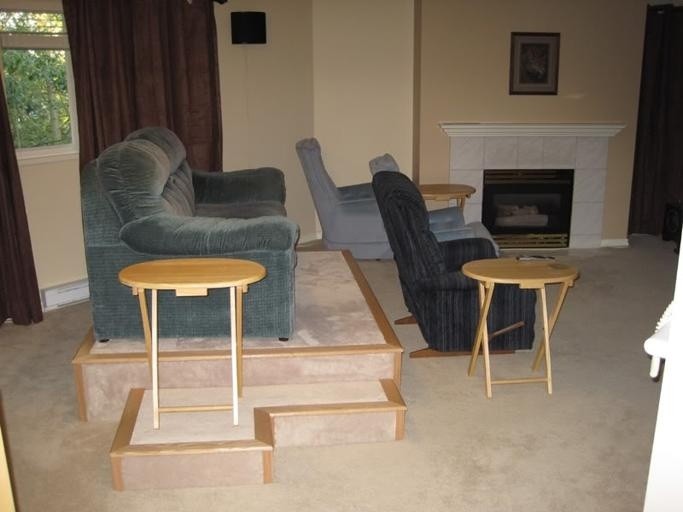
[508,30,560,95]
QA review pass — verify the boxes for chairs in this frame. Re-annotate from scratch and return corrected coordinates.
[296,138,393,262]
[368,153,502,258]
[371,171,538,358]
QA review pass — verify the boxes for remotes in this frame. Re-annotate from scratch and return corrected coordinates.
[518,256,557,260]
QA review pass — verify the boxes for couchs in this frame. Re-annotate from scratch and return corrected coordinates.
[79,124,300,343]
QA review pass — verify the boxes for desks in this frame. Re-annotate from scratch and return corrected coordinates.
[118,257,266,431]
[412,183,477,217]
[462,257,577,401]
